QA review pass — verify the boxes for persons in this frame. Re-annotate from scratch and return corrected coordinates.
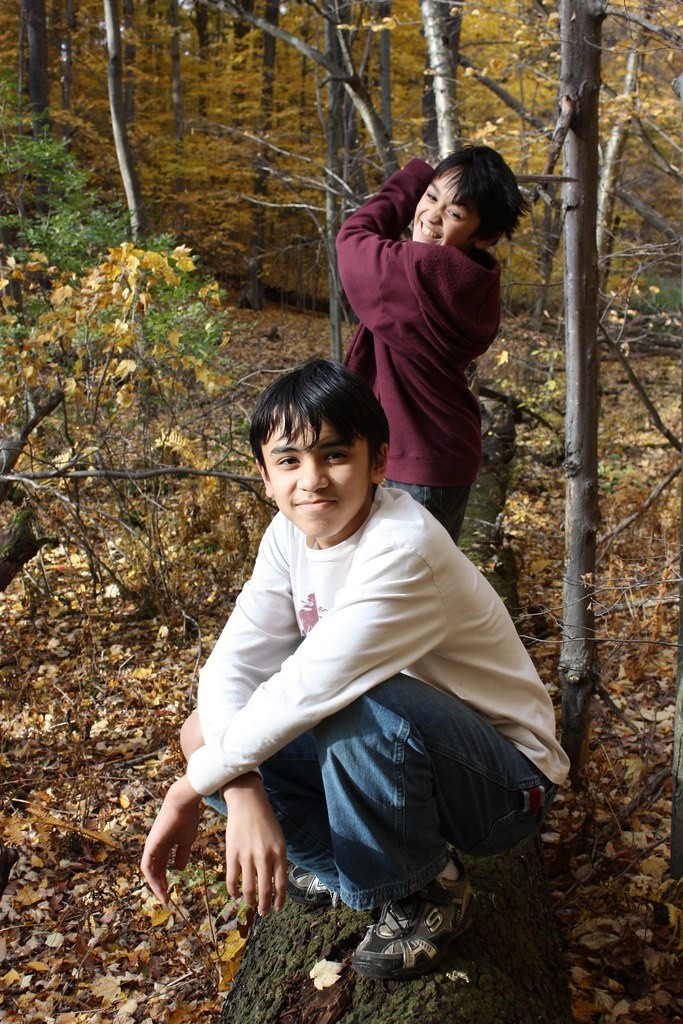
[139,358,571,982]
[335,144,537,547]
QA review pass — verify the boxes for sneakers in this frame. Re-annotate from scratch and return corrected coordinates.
[286,865,342,907]
[350,848,482,981]
